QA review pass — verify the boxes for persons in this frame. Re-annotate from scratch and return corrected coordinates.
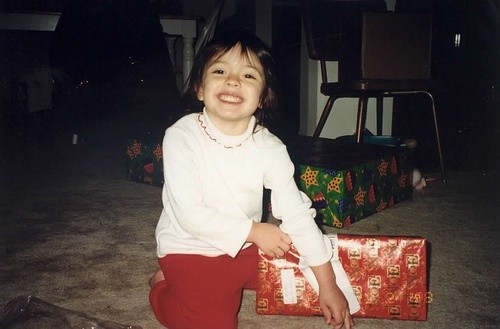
[149,29,355,329]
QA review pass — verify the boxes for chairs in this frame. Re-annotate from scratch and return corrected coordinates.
[305,18,447,184]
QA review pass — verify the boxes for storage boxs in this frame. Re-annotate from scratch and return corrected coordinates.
[256,235,428,322]
[121,111,413,229]
